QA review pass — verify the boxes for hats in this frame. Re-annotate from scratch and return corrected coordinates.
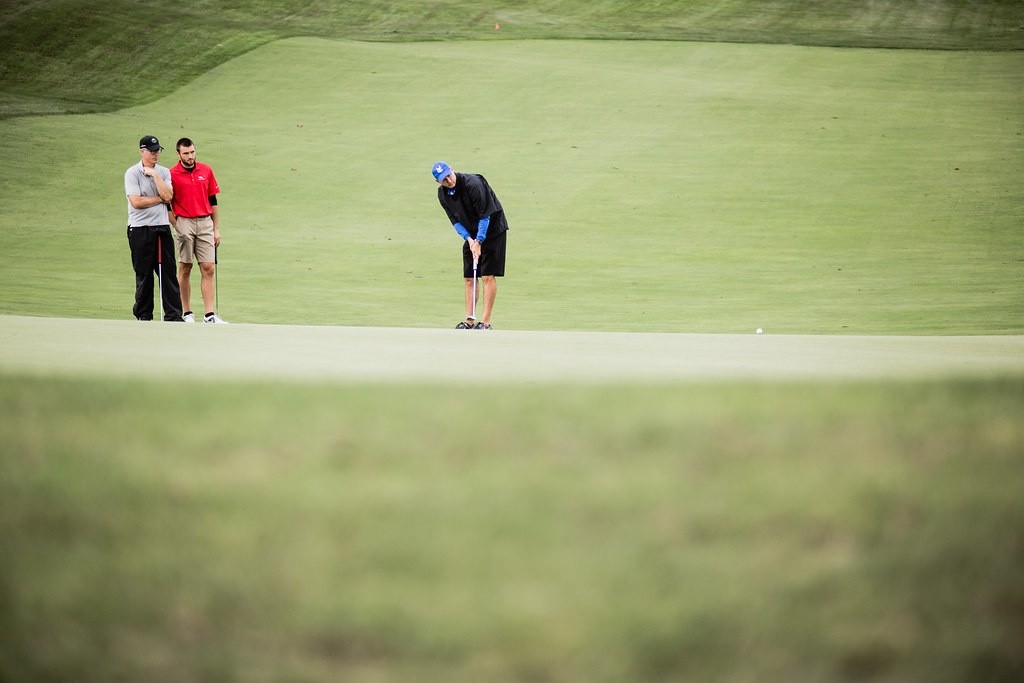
[432,162,451,182]
[139,135,164,150]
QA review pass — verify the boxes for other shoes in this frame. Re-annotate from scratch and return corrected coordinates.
[169,317,184,321]
[474,321,492,330]
[455,321,475,329]
[138,317,151,322]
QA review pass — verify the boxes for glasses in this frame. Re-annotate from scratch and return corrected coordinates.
[144,148,162,155]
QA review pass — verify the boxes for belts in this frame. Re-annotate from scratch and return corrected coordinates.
[196,215,209,218]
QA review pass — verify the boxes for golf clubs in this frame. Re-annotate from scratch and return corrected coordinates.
[467,239,478,321]
[158,234,164,321]
[214,243,223,320]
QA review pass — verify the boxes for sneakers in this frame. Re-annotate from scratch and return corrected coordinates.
[203,315,228,324]
[183,314,195,323]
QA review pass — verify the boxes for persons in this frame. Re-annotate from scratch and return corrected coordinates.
[166,138,228,323]
[124,135,185,321]
[432,163,509,329]
